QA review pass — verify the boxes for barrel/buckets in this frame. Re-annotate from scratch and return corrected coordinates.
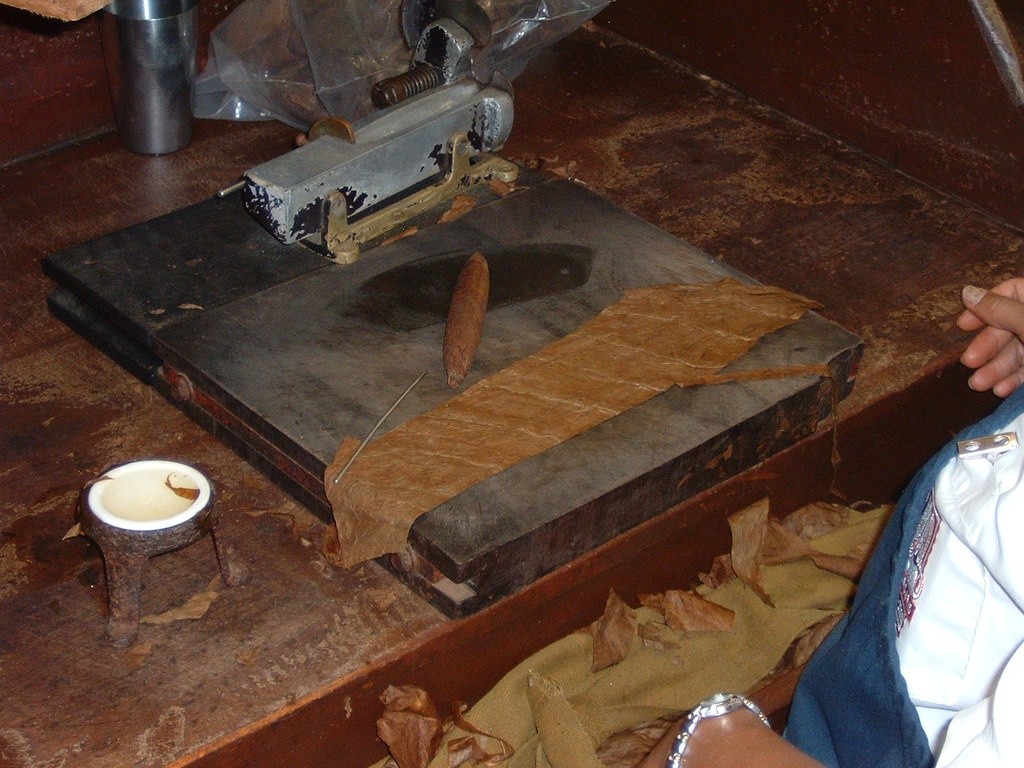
[100,0,201,156]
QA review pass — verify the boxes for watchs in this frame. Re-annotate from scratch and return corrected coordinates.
[666,691,770,768]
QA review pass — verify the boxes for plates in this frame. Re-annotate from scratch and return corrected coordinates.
[88,460,211,529]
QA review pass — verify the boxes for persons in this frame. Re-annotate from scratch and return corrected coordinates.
[635,275,1024,768]
